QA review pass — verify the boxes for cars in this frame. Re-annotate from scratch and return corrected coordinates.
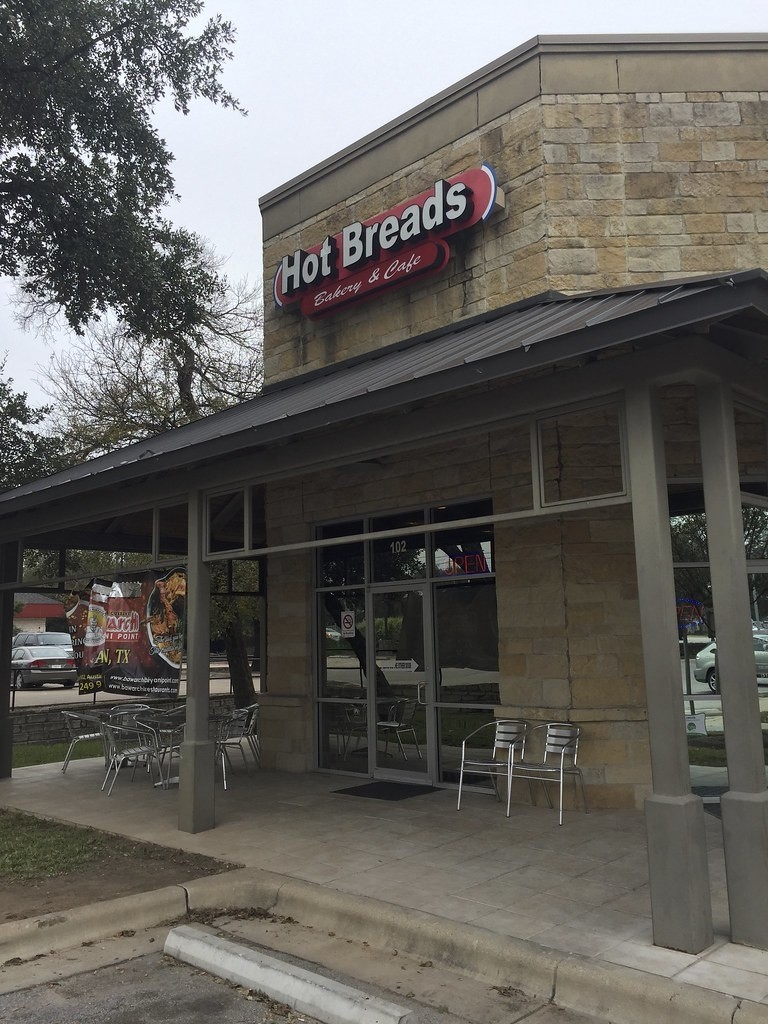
[692,635,768,693]
[326,627,341,640]
[11,647,77,688]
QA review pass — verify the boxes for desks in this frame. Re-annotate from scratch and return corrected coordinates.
[144,714,246,725]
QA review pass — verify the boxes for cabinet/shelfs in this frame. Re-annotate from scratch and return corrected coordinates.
[89,709,167,715]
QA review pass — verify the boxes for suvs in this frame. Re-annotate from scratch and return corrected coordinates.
[12,631,74,662]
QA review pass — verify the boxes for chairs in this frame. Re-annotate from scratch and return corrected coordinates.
[60,702,262,797]
[457,720,537,819]
[336,695,422,761]
[506,723,592,827]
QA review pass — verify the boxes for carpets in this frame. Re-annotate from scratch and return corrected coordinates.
[442,770,488,784]
[331,780,447,801]
